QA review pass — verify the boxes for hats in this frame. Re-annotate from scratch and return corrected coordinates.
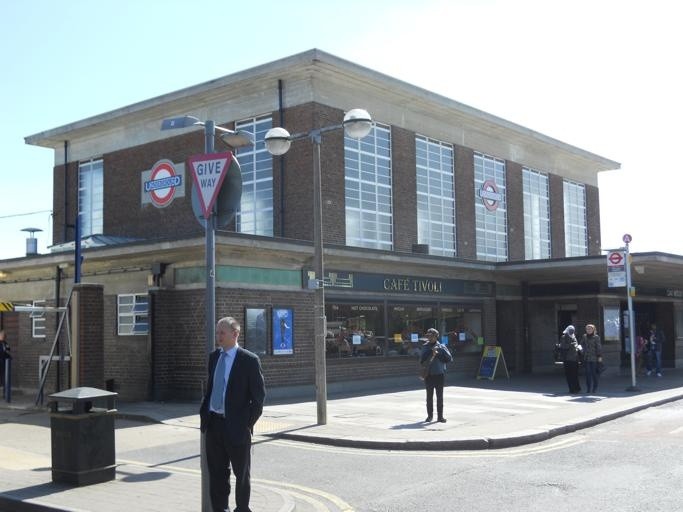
[425,327,439,337]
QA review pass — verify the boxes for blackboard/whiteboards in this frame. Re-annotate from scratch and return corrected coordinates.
[478,346,501,376]
[604,305,620,341]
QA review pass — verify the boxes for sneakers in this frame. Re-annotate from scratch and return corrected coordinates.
[646,370,651,376]
[656,372,661,377]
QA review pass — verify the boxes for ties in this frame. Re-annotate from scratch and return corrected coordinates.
[211,351,226,410]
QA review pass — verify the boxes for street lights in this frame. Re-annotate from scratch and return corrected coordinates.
[264,107,377,426]
[156,114,259,507]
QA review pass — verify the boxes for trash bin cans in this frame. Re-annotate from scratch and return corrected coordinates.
[47,386,118,487]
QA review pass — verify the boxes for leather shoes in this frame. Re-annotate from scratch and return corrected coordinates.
[438,417,446,423]
[425,416,432,421]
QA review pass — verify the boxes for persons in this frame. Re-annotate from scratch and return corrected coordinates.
[560,325,581,394]
[199,316,265,512]
[644,324,666,377]
[419,327,452,422]
[635,326,648,376]
[577,324,603,393]
[0,330,12,386]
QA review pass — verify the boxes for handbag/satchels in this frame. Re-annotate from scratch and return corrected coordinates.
[596,362,606,372]
[418,361,429,381]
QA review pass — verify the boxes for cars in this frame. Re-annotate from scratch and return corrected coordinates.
[324,327,428,357]
